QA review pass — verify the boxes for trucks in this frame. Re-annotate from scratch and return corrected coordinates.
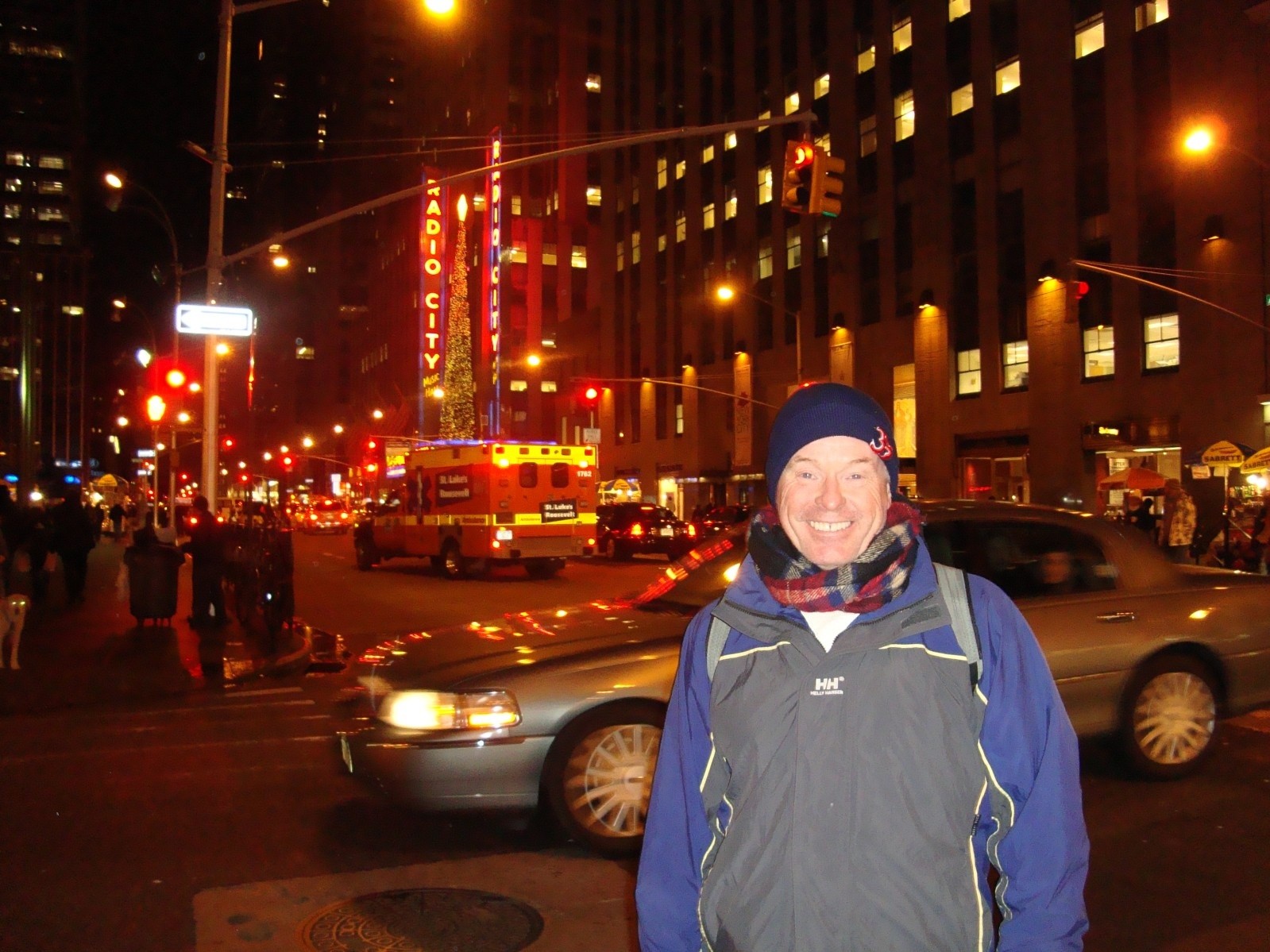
[353,440,599,579]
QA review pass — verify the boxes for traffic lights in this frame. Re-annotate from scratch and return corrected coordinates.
[585,383,599,410]
[1066,280,1088,323]
[782,141,812,217]
[810,147,844,220]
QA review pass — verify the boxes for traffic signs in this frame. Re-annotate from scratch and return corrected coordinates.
[175,305,252,337]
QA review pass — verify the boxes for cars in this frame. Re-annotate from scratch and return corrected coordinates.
[336,500,1270,861]
[584,502,759,561]
[172,498,371,535]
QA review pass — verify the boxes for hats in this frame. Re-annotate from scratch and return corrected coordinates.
[766,382,898,508]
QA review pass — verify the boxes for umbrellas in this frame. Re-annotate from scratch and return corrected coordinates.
[1099,467,1167,491]
[94,474,129,488]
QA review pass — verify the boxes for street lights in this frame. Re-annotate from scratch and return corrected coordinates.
[111,295,158,360]
[717,287,802,390]
[202,1,457,512]
[102,166,180,540]
[145,395,164,526]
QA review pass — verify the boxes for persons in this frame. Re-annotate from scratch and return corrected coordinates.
[1162,478,1196,564]
[0,483,229,625]
[636,384,1089,952]
[1132,498,1157,534]
